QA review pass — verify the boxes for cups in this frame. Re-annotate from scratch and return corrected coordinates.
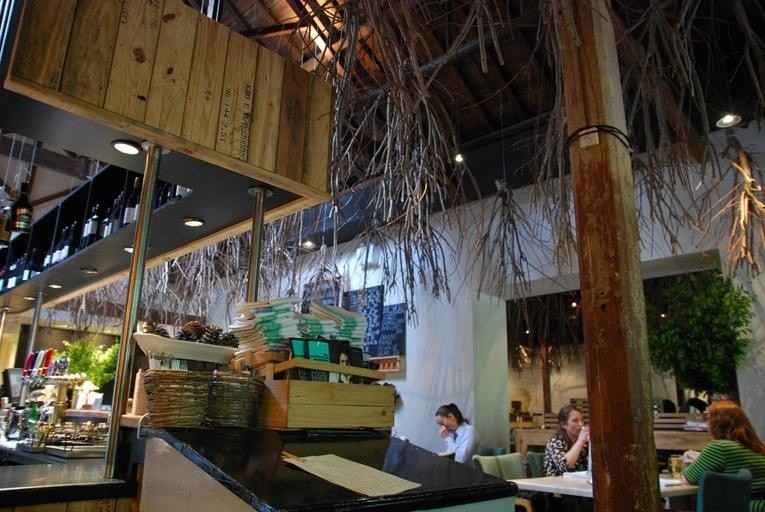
[671,455,683,480]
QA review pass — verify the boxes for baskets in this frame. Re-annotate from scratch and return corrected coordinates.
[209,366,267,429]
[142,364,215,431]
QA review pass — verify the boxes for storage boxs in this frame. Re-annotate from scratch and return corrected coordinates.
[225,352,396,430]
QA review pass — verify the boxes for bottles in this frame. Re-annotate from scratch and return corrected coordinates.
[0,177,184,294]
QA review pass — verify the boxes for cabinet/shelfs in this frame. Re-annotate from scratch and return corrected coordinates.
[515,413,713,460]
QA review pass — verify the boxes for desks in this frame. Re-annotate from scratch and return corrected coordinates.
[505,470,696,512]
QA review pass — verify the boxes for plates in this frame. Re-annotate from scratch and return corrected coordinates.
[133,333,238,364]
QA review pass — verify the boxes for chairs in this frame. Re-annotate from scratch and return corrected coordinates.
[695,469,753,512]
[472,451,546,481]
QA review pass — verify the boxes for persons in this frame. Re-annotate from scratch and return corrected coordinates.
[434,402,480,468]
[542,403,593,511]
[335,344,361,384]
[682,399,765,512]
